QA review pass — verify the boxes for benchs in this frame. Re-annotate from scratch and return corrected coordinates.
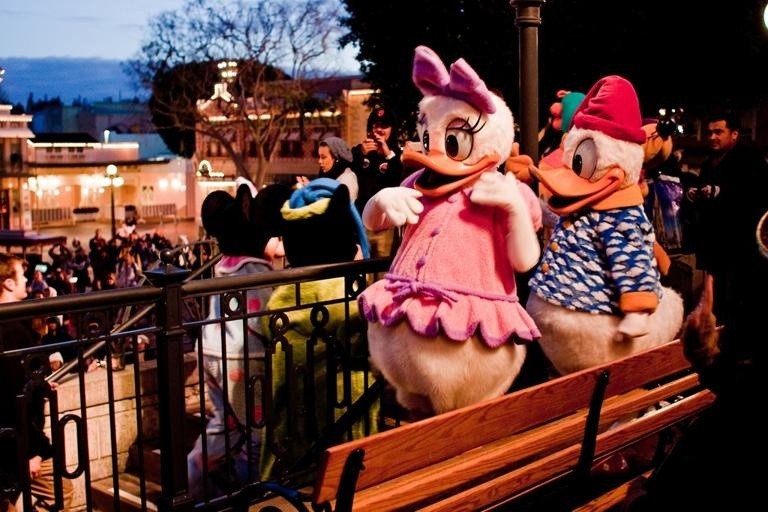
[263,323,729,511]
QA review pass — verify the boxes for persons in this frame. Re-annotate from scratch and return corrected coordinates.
[297,94,398,201]
[0,205,217,512]
[684,107,768,355]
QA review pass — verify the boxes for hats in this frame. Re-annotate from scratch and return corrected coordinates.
[320,136,353,163]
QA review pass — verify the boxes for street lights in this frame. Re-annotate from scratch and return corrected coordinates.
[100,164,124,244]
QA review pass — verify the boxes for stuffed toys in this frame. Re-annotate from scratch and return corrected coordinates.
[189,180,380,495]
[349,46,683,416]
[503,74,683,417]
[357,46,543,416]
